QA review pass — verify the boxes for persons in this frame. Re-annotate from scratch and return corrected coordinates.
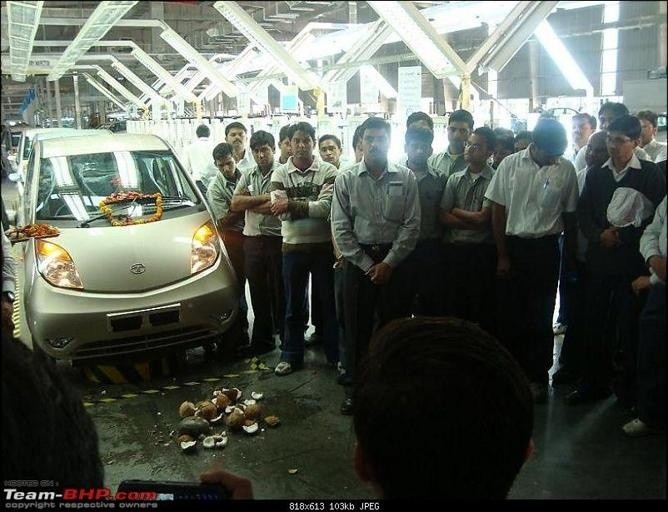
[1,329,124,499]
[1,221,17,323]
[181,101,666,498]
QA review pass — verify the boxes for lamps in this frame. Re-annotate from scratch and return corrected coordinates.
[1,1,598,115]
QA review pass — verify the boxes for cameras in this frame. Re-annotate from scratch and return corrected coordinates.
[118,480,229,500]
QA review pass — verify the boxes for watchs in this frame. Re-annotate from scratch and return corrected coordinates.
[3,290,16,303]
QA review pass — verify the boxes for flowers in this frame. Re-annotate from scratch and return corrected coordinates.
[97,187,165,227]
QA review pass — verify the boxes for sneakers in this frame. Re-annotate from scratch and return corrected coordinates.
[623,419,662,437]
[340,396,354,416]
[564,386,611,405]
[532,383,551,404]
[275,357,300,375]
